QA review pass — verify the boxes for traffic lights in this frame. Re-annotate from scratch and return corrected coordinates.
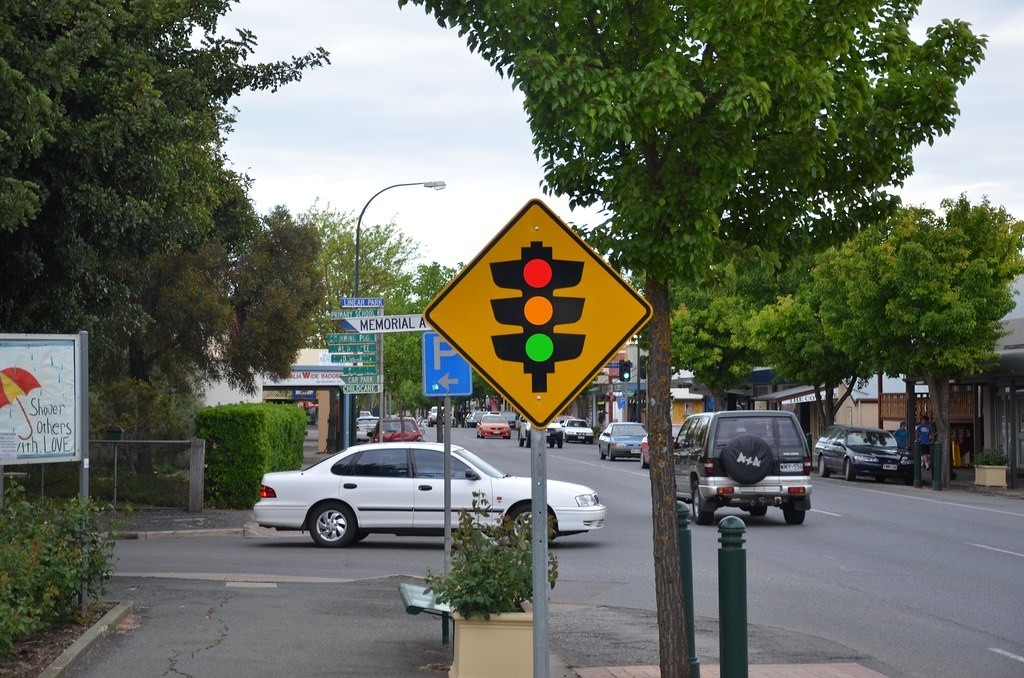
[622,361,630,381]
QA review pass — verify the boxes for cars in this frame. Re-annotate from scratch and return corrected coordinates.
[254,442,607,548]
[464,411,487,428]
[477,414,513,440]
[414,418,428,427]
[556,417,595,444]
[597,422,684,469]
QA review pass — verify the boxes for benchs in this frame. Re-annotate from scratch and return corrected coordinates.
[397,582,457,644]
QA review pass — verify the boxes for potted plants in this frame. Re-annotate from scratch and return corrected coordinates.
[973,444,1011,487]
[440,501,551,678]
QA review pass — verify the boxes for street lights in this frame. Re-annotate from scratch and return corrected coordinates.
[349,181,446,446]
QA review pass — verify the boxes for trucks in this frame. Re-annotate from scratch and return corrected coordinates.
[488,411,516,428]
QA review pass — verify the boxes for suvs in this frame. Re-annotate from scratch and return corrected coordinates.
[367,417,425,442]
[355,417,380,442]
[672,410,812,524]
[815,424,914,482]
[428,406,452,427]
[359,411,373,417]
[518,414,564,448]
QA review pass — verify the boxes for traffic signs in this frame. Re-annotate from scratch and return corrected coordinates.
[326,297,433,394]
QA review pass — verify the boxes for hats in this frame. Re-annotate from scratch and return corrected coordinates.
[900,420,906,426]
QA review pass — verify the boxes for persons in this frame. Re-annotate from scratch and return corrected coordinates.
[893,420,907,449]
[916,415,935,470]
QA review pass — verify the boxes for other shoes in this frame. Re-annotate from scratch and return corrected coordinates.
[926,466,931,470]
[923,464,926,469]
